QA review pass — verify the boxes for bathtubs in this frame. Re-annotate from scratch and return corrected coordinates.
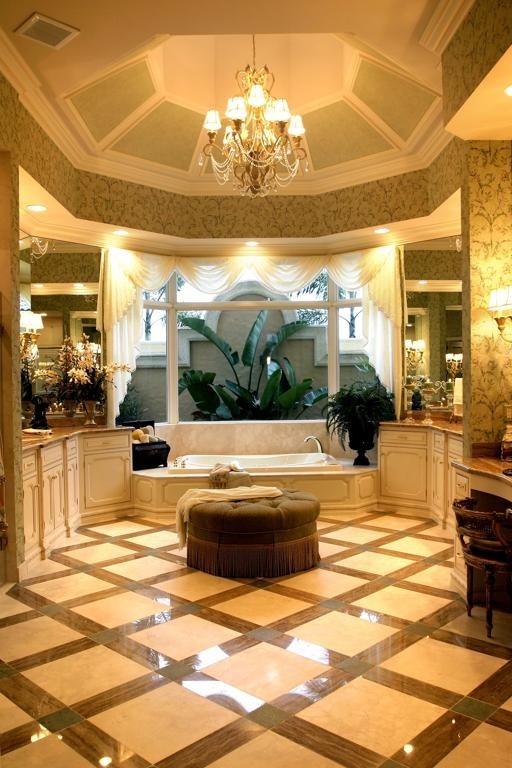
[168,453,344,473]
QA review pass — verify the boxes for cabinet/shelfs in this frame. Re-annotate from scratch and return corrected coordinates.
[20,450,43,562]
[378,425,432,517]
[65,436,82,537]
[450,466,511,608]
[428,430,465,534]
[83,431,137,525]
[39,441,67,550]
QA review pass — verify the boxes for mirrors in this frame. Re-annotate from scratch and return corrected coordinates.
[401,235,465,413]
[18,224,107,430]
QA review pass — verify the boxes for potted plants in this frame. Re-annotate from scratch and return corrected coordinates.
[321,378,397,465]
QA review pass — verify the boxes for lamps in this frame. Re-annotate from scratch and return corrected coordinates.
[201,37,310,199]
[445,353,463,378]
[486,287,512,341]
[406,337,427,371]
[19,309,46,357]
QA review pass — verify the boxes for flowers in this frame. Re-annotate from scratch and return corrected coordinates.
[31,360,59,392]
[59,331,134,403]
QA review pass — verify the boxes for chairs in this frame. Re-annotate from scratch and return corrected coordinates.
[452,495,512,636]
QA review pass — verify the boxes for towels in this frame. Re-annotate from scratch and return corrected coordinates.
[175,486,283,551]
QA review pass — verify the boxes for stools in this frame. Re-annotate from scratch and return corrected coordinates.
[185,486,322,578]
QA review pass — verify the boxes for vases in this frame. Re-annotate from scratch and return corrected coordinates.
[79,398,101,428]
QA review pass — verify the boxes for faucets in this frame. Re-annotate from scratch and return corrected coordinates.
[304,436,322,453]
